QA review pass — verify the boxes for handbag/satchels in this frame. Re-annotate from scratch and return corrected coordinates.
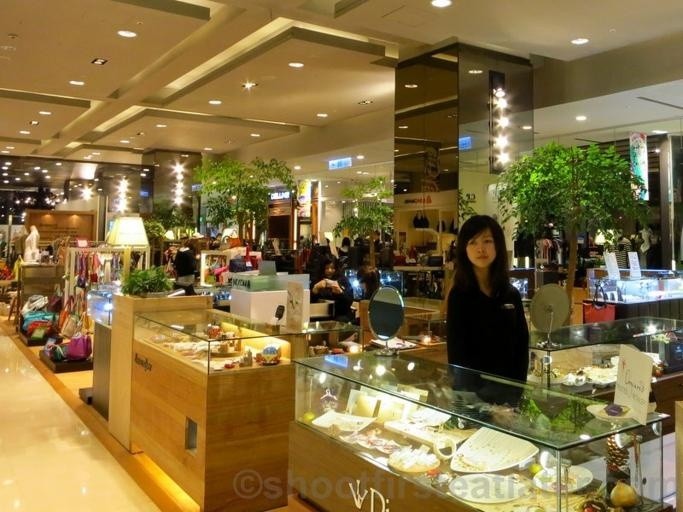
[45,337,67,363]
[20,294,62,340]
[58,310,70,329]
[60,315,80,339]
[68,334,92,358]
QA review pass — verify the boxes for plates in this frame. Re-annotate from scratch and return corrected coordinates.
[449,473,531,505]
[390,445,439,473]
[310,408,378,433]
[448,425,540,476]
[410,404,453,428]
[586,402,636,424]
[533,464,594,495]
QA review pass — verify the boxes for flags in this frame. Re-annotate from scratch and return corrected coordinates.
[627,130,649,203]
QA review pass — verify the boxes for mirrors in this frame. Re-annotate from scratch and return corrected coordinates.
[368,284,404,357]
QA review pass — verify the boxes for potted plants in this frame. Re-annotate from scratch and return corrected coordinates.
[120,262,176,298]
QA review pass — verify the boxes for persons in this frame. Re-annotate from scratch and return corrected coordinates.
[22,225,40,261]
[0,233,6,259]
[446,215,529,428]
[373,229,382,252]
[311,252,355,342]
[216,233,221,239]
[338,237,351,256]
[172,247,196,286]
